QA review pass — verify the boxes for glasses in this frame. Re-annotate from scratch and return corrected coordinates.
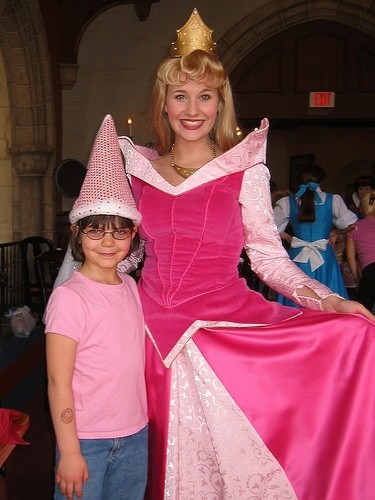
[75,224,133,241]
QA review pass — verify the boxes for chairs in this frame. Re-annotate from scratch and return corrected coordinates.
[32,246,69,307]
[20,234,57,318]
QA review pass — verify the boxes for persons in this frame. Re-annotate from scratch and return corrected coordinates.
[44,113,149,500]
[115,7,375,500]
[273,163,359,308]
[346,186,375,316]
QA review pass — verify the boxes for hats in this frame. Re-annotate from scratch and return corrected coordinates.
[69,114,142,228]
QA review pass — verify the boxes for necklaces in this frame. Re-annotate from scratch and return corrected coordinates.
[171,138,216,178]
